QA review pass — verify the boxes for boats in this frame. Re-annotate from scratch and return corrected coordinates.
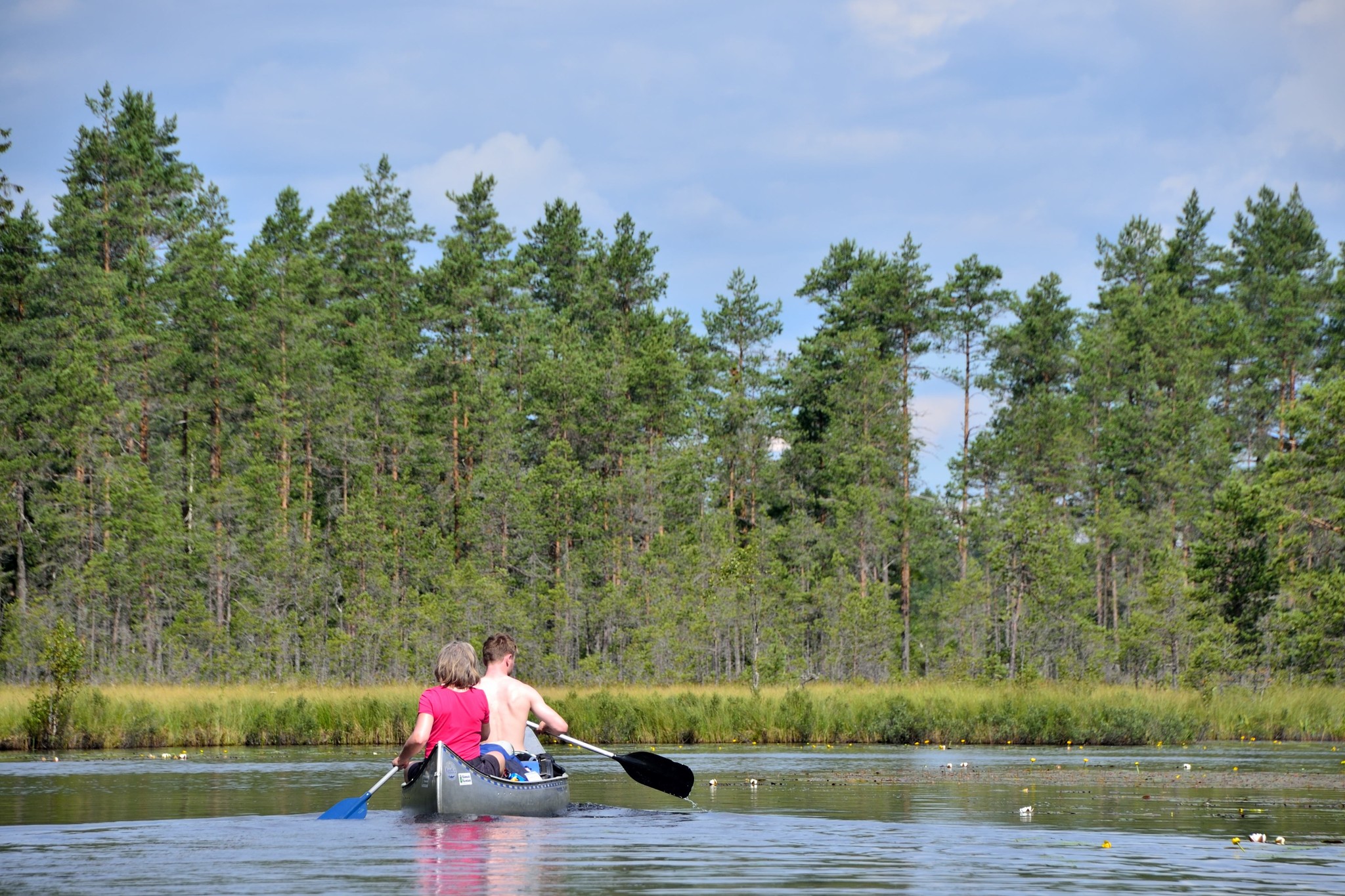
[401,726,570,816]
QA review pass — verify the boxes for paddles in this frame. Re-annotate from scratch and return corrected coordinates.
[526,721,694,799]
[316,766,401,820]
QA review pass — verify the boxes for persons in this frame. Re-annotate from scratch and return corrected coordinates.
[471,634,569,762]
[391,641,505,784]
